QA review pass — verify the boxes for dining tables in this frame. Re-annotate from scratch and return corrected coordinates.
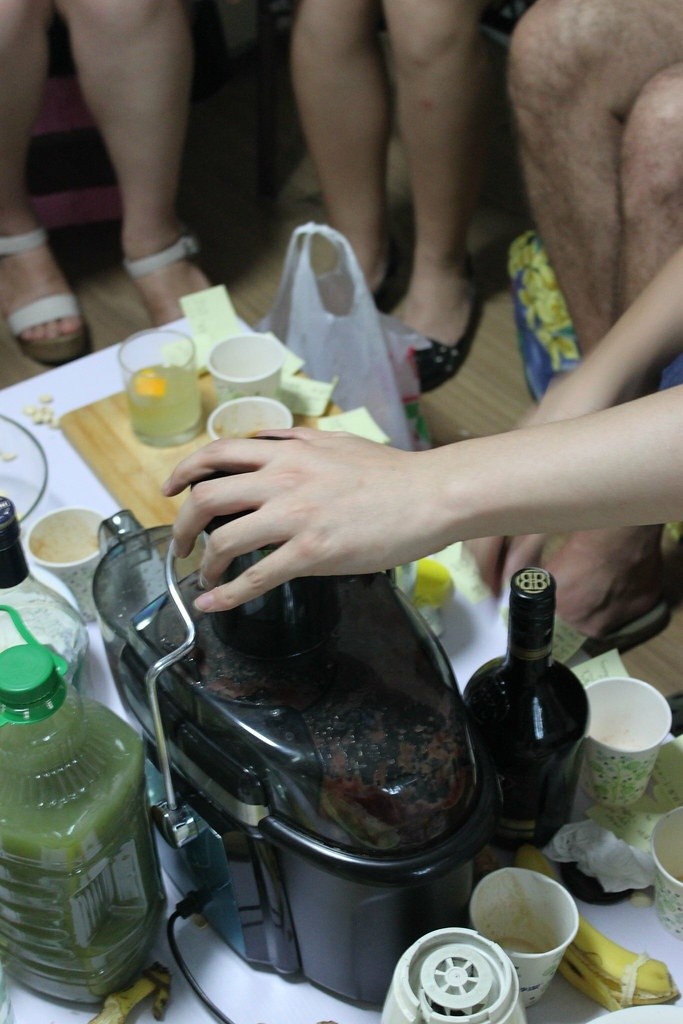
[0,313,683,1024]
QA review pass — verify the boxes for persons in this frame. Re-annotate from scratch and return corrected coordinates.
[1,0,216,361]
[258,0,483,396]
[505,0,683,667]
[161,245,683,611]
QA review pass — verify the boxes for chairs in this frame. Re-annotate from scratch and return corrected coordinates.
[509,231,683,736]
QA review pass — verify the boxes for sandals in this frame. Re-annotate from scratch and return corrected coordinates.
[0,230,91,366]
[125,235,215,329]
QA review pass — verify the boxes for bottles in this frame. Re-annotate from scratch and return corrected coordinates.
[464,565,590,849]
[0,497,88,693]
[1,604,168,1004]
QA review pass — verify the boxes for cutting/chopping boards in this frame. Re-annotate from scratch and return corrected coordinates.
[60,366,344,581]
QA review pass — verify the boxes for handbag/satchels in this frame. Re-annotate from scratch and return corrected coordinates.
[258,222,433,452]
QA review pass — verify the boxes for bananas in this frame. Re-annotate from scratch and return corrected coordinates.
[509,840,679,1013]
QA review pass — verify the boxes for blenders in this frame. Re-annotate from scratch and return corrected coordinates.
[92,509,494,1004]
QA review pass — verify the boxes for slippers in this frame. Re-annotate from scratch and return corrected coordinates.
[584,600,672,658]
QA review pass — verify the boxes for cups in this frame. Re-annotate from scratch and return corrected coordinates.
[469,867,581,1008]
[206,333,281,402]
[586,676,672,807]
[650,805,683,939]
[206,396,294,442]
[27,507,104,624]
[117,329,205,448]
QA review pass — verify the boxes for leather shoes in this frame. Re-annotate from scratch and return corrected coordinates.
[374,242,404,307]
[414,270,482,394]
